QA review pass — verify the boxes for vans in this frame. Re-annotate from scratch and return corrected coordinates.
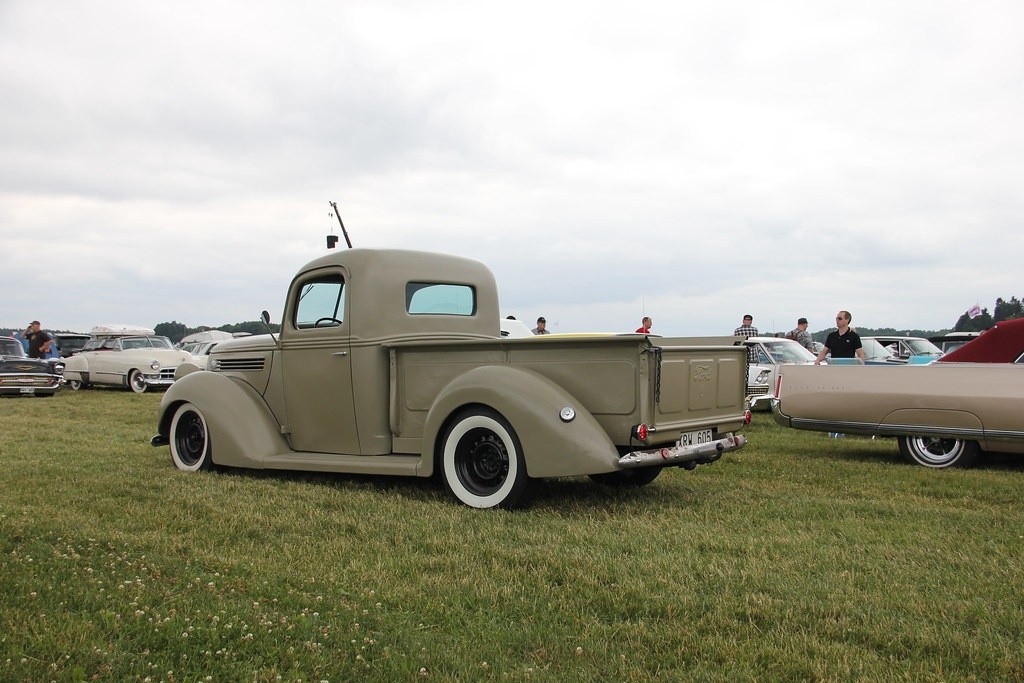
[810,330,984,364]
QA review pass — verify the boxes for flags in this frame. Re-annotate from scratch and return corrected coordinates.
[968,304,982,320]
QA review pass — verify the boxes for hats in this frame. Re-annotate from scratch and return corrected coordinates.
[30,321,40,325]
[798,318,807,324]
[743,315,752,321]
[537,317,546,323]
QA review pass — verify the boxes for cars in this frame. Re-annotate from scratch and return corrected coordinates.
[174,330,253,370]
[49,333,92,358]
[743,337,828,411]
[0,336,68,397]
[63,332,205,393]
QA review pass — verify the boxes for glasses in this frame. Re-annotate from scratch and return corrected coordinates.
[836,316,849,321]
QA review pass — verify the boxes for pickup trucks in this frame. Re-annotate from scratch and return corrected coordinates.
[769,317,1024,468]
[151,249,752,513]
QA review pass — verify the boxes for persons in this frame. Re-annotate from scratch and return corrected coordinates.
[775,333,780,338]
[21,320,53,359]
[785,318,813,353]
[814,311,864,365]
[636,317,651,333]
[531,317,550,336]
[734,315,758,361]
